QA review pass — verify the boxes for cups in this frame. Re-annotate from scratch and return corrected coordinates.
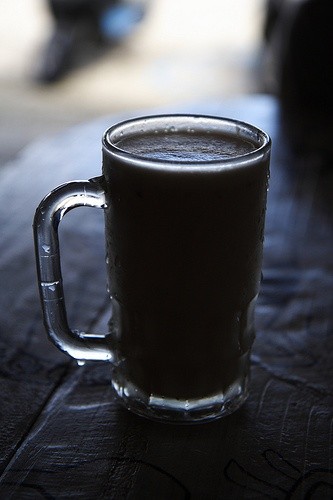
[32,113,271,425]
